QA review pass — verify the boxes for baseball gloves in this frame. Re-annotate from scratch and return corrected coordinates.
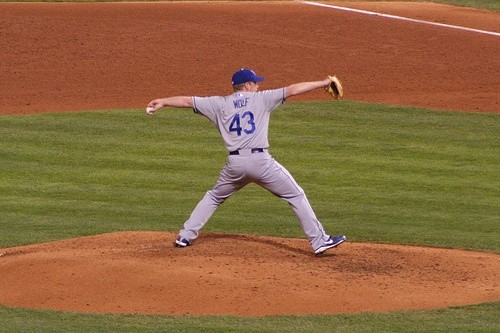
[328,76,344,101]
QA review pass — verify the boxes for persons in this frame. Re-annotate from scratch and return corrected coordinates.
[147,67,348,256]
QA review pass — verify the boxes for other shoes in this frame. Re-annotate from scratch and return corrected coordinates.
[175,236,192,246]
[315,235,347,254]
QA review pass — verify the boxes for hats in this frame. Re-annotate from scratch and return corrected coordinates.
[231,68,264,84]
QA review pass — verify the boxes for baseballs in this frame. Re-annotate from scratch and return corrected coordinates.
[145,107,154,115]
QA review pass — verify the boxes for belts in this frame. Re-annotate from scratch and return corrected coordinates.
[230,147,263,154]
